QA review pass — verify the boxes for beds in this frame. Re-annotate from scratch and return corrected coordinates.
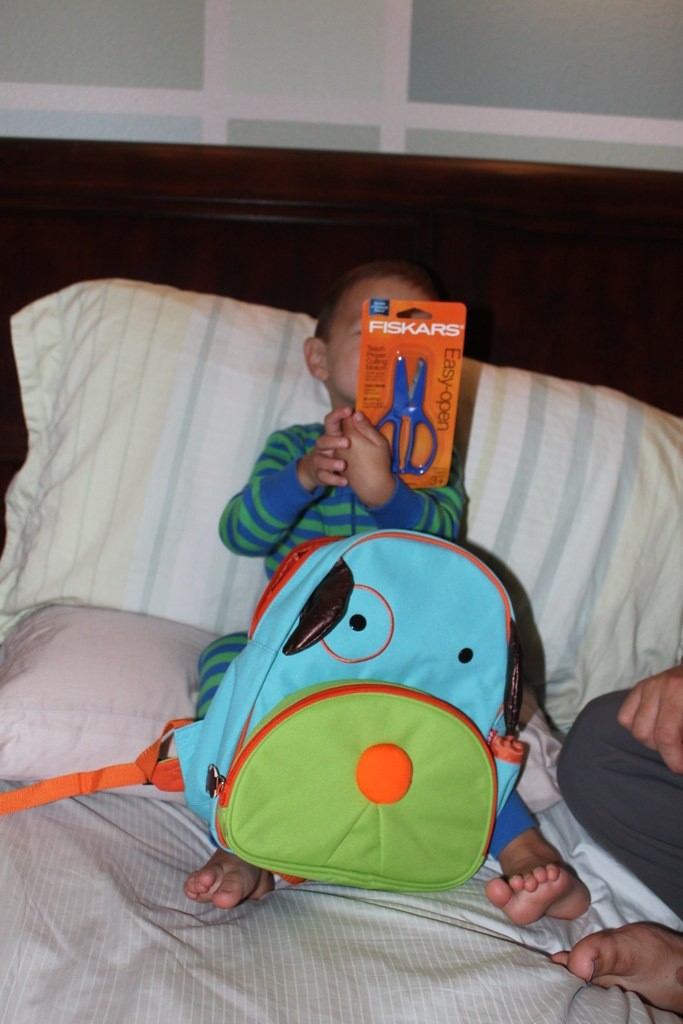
[0,135,683,1024]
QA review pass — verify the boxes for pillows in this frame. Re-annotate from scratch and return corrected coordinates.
[0,274,683,733]
[0,603,228,803]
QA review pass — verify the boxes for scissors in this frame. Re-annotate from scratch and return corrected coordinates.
[372,352,439,477]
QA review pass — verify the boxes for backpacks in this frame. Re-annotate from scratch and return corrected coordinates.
[0,529,525,894]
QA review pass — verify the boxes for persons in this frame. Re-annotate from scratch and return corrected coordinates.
[185,261,591,923]
[551,649,683,1015]
